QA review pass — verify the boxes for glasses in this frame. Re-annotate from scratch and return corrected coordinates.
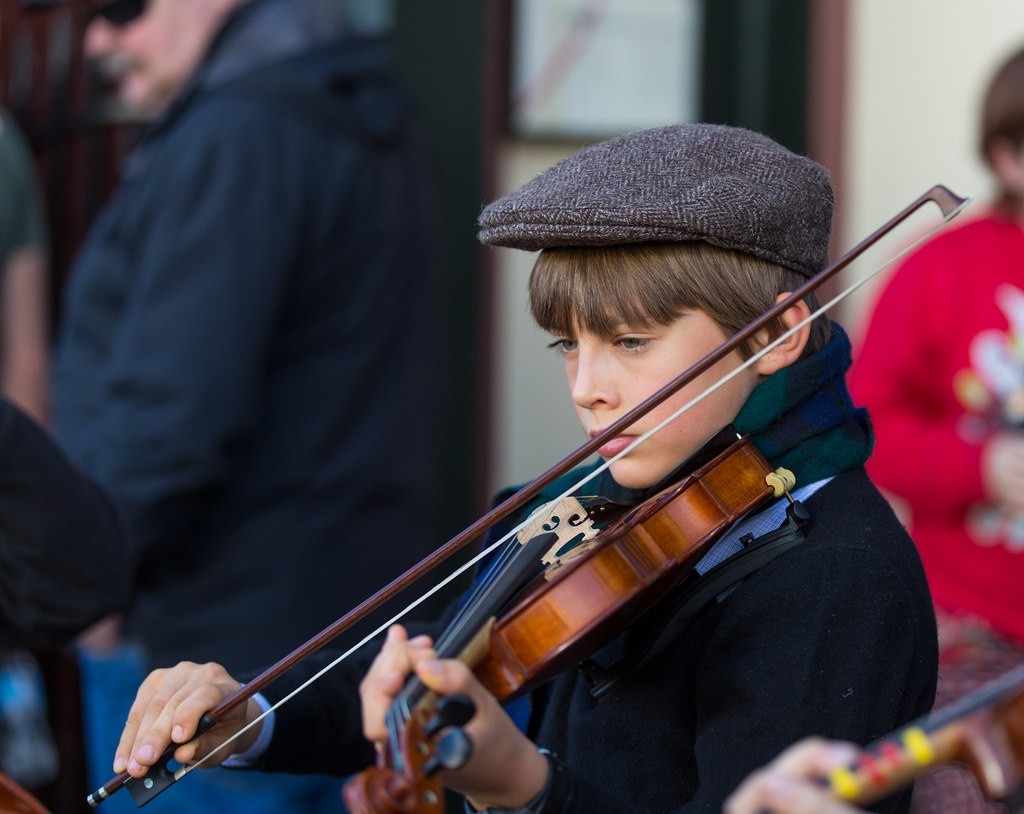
[68,0,146,39]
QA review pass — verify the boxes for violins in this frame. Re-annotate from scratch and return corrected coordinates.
[343,438,796,814]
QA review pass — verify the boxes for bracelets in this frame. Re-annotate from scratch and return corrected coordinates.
[464,747,560,813]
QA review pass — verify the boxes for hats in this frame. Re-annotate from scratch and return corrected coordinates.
[476,124,835,279]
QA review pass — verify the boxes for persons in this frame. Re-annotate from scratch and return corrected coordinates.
[0,0,1024,813]
